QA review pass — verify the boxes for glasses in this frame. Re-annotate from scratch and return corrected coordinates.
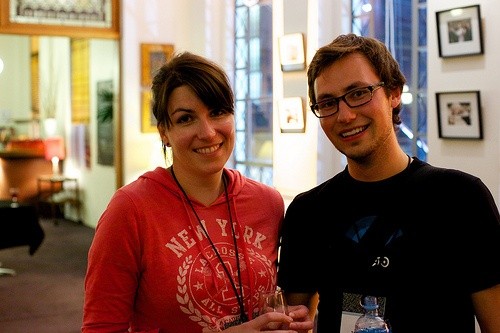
[310,81,385,118]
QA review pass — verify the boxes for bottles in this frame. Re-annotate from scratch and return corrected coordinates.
[353,297,392,333]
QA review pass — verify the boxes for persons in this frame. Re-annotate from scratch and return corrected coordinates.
[277,33,500,333]
[80,52,314,333]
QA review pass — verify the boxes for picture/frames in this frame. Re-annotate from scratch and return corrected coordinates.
[141,92,158,132]
[140,43,174,87]
[0,0,121,40]
[436,4,484,58]
[435,90,483,140]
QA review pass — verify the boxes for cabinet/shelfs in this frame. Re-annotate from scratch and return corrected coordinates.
[37,175,82,226]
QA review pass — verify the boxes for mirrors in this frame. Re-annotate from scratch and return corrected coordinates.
[96,80,115,165]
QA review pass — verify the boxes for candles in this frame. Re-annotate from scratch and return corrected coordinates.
[51,157,59,175]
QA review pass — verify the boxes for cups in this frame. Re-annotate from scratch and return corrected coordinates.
[258,290,290,330]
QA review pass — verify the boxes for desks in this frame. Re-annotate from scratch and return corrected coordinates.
[0,152,62,203]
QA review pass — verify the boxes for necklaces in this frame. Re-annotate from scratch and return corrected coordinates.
[170,164,250,323]
